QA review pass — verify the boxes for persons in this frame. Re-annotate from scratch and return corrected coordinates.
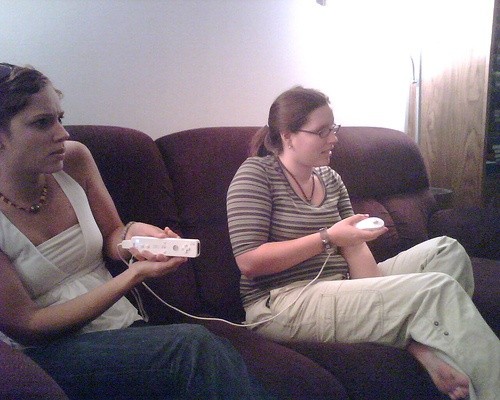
[1,63,347,399]
[226,88,500,400]
[0,339,67,399]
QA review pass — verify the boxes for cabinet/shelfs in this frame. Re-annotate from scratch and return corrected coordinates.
[406,0,499,207]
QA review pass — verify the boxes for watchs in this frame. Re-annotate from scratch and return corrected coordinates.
[320,229,337,255]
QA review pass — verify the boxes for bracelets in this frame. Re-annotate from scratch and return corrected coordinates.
[121,221,134,240]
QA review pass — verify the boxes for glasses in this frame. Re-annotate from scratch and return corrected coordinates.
[293,123,342,138]
[1,62,15,81]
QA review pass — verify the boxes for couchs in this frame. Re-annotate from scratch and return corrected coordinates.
[1,124,500,399]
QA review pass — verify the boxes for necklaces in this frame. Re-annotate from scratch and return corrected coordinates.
[0,184,49,214]
[277,156,315,204]
